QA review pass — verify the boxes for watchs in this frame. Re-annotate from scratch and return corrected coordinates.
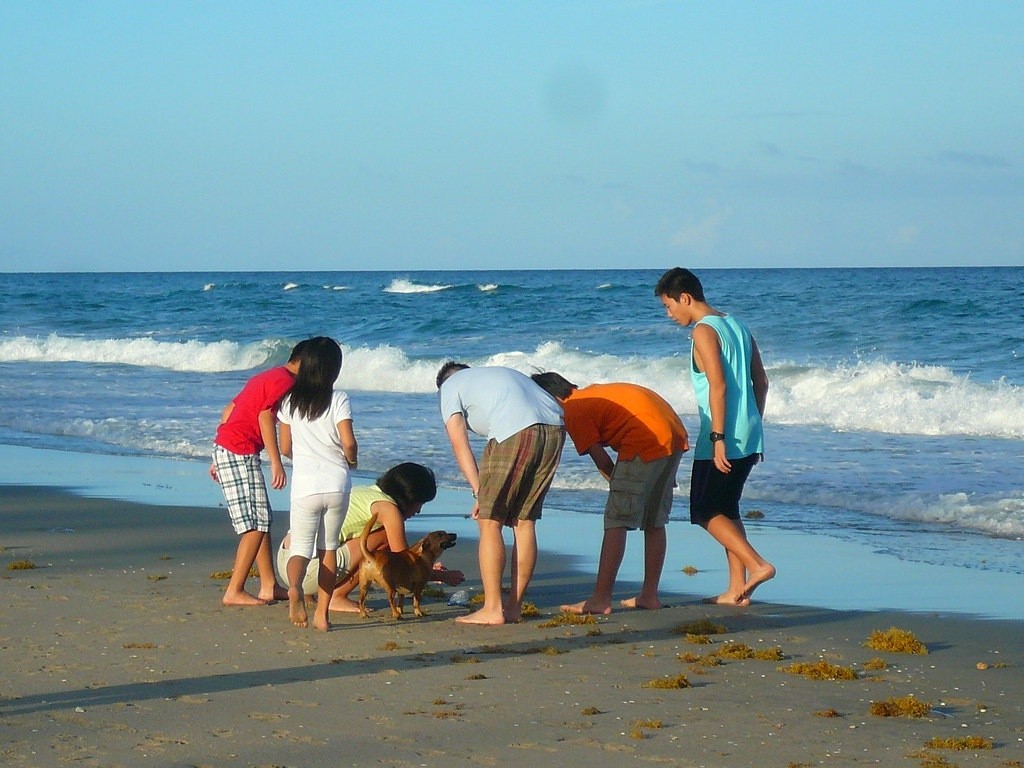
[709,431,726,442]
[472,491,479,499]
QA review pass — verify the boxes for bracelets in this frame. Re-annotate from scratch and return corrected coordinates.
[346,459,358,465]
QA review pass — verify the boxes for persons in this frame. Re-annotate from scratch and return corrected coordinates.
[277,461,465,614]
[210,340,308,606]
[436,362,567,625]
[529,373,690,615]
[276,337,357,632]
[655,267,777,606]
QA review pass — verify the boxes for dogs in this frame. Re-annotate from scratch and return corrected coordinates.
[358,512,457,619]
